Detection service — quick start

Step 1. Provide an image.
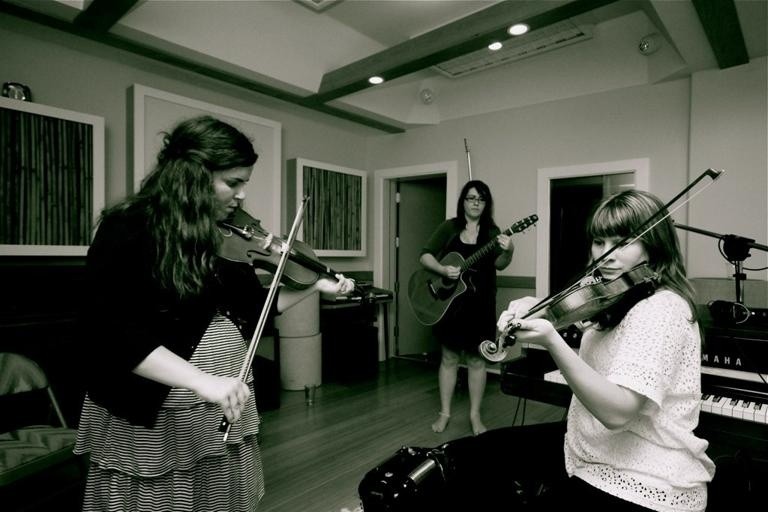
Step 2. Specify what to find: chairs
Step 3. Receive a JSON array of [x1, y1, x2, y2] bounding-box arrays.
[[1, 352, 76, 511]]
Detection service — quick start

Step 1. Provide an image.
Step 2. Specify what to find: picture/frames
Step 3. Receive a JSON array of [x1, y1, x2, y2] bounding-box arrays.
[[126, 85, 281, 238], [1, 97, 105, 255], [286, 157, 365, 255]]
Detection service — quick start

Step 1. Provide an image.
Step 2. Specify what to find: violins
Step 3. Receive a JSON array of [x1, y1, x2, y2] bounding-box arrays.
[[215, 205, 377, 313], [476, 260, 658, 365]]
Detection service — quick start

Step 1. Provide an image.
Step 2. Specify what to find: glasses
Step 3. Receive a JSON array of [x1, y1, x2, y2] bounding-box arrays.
[[465, 194, 487, 204]]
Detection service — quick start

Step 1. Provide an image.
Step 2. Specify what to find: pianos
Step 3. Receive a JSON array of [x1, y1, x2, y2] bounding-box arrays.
[[499, 301, 763, 496]]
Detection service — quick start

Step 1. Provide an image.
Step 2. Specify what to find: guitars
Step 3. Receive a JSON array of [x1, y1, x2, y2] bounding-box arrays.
[[406, 214, 538, 325]]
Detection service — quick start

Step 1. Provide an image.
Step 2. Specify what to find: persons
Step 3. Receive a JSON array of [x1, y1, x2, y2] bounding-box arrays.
[[446, 189, 719, 512], [418, 178, 514, 436], [70, 115, 355, 511]]
